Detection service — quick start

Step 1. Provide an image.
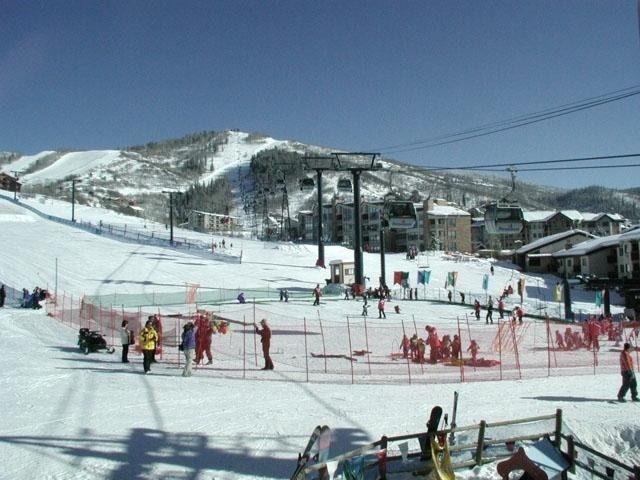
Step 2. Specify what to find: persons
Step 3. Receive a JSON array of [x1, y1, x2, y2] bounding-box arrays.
[[488, 295, 494, 308], [19, 286, 48, 310], [151, 317, 157, 331], [555, 312, 640, 352], [515, 306, 523, 323], [155, 314, 162, 354], [0, 284, 7, 307], [344, 283, 418, 319], [177, 309, 214, 377], [121, 320, 135, 363], [617, 343, 639, 402], [237, 292, 247, 303], [485, 303, 494, 325], [473, 301, 481, 321], [459, 291, 465, 304], [312, 283, 323, 306], [447, 290, 453, 304], [254, 318, 275, 370], [496, 296, 505, 319], [405, 245, 420, 260], [140, 320, 157, 374], [398, 325, 480, 364]]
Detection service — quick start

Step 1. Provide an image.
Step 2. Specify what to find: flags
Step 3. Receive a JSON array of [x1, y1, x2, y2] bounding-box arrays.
[[555, 284, 562, 303], [596, 290, 603, 307], [394, 270, 459, 287], [482, 274, 490, 289]]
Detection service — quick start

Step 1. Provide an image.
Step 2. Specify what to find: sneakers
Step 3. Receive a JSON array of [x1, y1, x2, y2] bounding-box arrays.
[[193, 358, 201, 363], [269, 365, 274, 369], [205, 360, 213, 365], [261, 366, 270, 370], [145, 369, 153, 375], [618, 397, 627, 403], [631, 397, 640, 402]]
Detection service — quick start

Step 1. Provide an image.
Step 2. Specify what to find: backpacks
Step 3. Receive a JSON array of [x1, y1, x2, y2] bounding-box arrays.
[[126, 328, 135, 345]]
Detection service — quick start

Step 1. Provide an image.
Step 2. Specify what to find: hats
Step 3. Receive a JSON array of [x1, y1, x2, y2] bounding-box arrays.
[[258, 319, 267, 323]]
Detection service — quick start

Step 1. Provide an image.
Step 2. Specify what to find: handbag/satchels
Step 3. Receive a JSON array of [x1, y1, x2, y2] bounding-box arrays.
[[179, 343, 183, 351]]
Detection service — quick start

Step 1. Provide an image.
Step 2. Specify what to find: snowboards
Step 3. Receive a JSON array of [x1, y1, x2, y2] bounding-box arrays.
[[291, 425, 331, 480], [413, 406, 442, 476]]
